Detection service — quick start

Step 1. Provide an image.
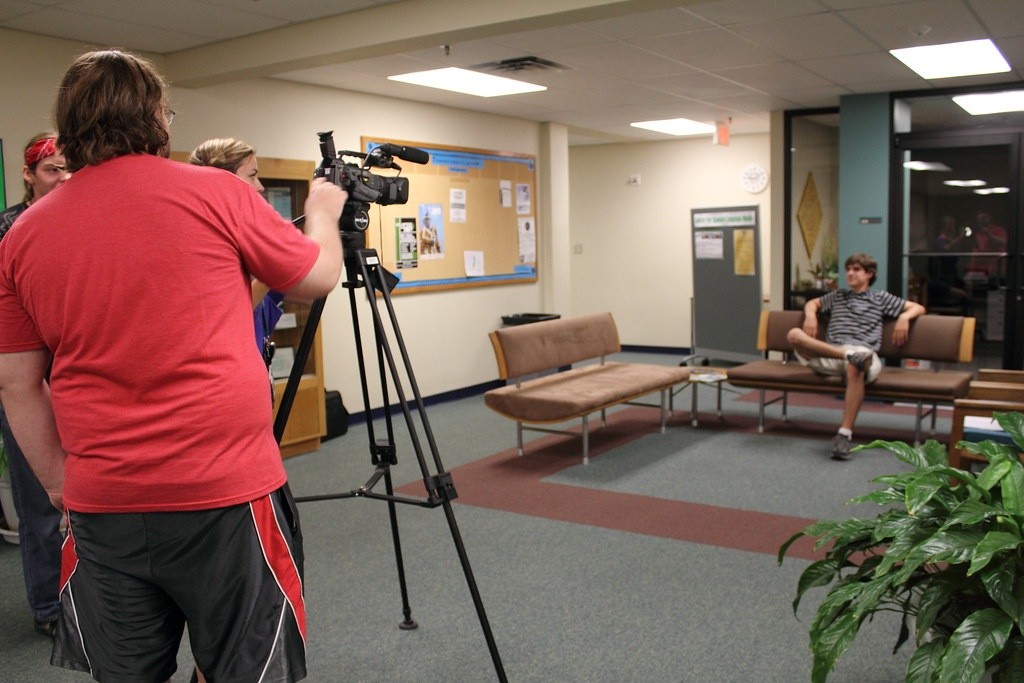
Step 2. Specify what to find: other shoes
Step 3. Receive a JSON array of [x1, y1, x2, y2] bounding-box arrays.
[[35, 615, 63, 637]]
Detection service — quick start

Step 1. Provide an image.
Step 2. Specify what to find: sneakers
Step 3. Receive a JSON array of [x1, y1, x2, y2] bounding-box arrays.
[[829, 431, 851, 460], [847, 349, 872, 373]]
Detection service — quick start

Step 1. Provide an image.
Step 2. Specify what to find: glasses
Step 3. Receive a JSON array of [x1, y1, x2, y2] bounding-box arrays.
[[159, 105, 176, 125]]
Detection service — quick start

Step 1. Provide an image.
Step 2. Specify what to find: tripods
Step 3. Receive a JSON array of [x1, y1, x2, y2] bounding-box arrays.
[[271, 200, 510, 683]]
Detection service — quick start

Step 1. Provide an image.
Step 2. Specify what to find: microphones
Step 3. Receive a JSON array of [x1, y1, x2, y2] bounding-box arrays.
[[380, 143, 429, 165]]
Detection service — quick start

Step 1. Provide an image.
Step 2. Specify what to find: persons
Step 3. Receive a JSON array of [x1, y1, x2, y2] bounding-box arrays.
[[915, 213, 1007, 307], [787, 254, 925, 459], [0, 51, 348, 682]]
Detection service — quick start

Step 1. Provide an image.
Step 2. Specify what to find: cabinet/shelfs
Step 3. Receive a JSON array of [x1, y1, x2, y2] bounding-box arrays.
[[170, 149, 328, 459]]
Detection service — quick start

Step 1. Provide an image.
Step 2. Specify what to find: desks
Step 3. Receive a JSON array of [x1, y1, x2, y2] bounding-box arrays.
[[949, 368, 1024, 485]]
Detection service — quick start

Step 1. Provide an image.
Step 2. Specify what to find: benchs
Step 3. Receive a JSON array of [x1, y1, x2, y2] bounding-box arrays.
[[727, 311, 975, 452], [486, 310, 691, 465]]
